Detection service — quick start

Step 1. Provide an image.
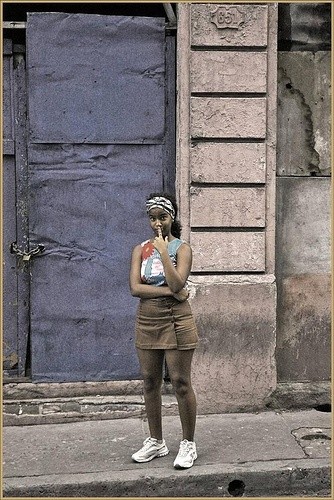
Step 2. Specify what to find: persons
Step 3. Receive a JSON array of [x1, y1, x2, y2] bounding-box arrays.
[[129, 191, 200, 470]]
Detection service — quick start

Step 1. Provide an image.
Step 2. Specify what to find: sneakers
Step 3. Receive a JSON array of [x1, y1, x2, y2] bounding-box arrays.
[[131, 437, 169, 463], [172, 439, 198, 469]]
